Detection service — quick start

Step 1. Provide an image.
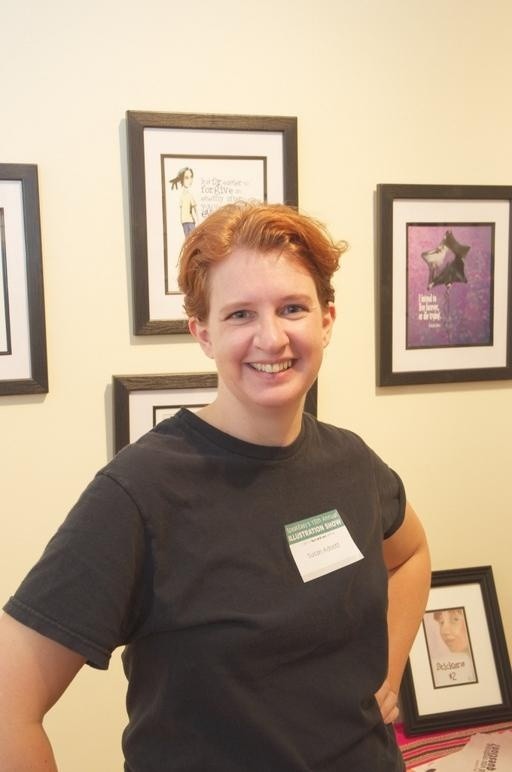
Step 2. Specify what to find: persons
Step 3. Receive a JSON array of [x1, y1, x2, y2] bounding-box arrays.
[[0, 196, 434, 772], [434, 608, 470, 653], [166, 163, 201, 237]]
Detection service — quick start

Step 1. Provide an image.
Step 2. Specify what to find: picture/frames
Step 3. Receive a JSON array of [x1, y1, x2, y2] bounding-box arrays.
[[374, 184, 510, 392], [1, 161, 49, 398], [125, 110, 301, 339], [113, 370, 319, 461], [396, 563, 512, 735]]
